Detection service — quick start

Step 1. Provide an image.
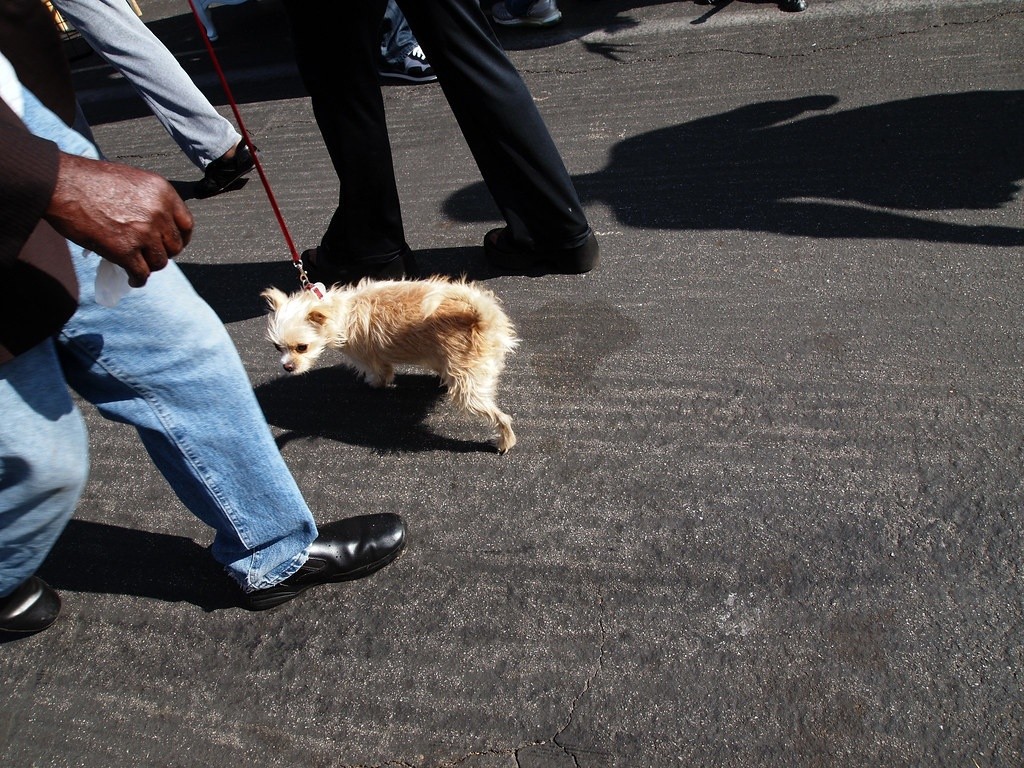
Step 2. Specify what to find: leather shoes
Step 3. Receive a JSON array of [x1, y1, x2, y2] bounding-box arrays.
[[192, 139, 265, 196], [230, 513, 406, 609], [0, 576, 63, 634]]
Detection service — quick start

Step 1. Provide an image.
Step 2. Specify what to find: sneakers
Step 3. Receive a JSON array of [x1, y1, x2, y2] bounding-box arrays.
[[378, 44, 438, 82], [491, 0, 561, 26]]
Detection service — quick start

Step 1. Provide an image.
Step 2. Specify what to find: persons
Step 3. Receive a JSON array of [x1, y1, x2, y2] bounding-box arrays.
[[0, 0, 408, 639], [284, 0, 599, 282], [490, 0, 562, 26], [377, 0, 437, 83], [50, 0, 259, 199]]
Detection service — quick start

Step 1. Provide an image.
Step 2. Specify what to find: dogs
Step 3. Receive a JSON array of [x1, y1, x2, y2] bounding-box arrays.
[[260, 271, 518, 454]]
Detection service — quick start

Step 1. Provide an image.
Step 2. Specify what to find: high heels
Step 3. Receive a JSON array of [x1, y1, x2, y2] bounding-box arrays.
[[484, 226, 601, 275], [302, 243, 416, 281]]
